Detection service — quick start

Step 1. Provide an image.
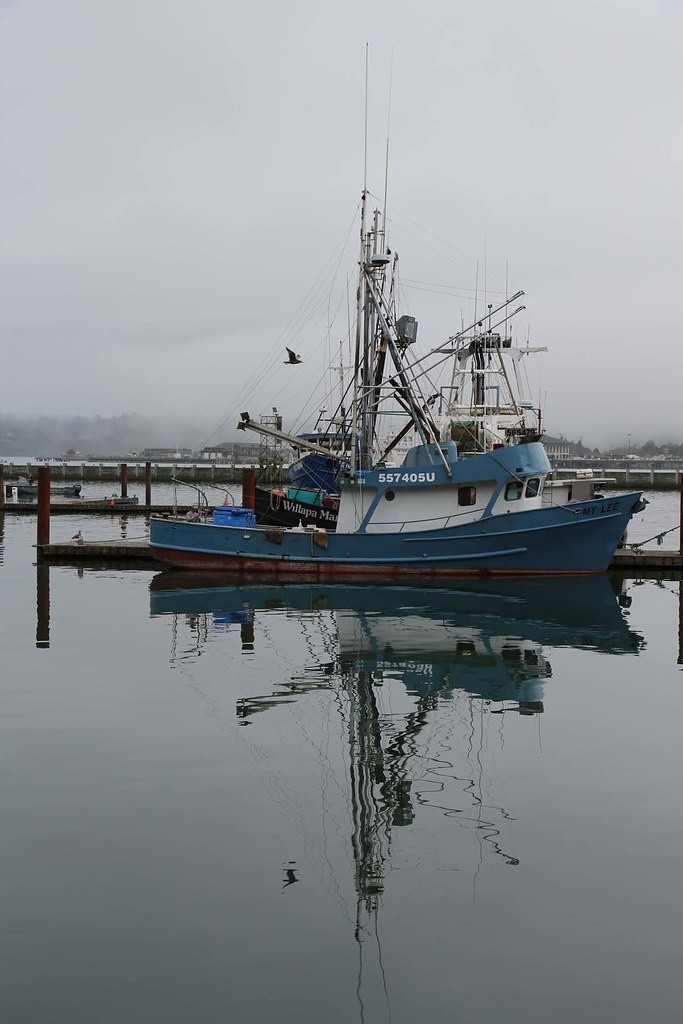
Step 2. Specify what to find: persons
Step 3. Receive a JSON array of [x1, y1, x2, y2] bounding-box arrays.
[[186, 504, 201, 523]]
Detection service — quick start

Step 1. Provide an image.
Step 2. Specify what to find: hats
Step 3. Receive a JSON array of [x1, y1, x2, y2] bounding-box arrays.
[[193, 504, 198, 509]]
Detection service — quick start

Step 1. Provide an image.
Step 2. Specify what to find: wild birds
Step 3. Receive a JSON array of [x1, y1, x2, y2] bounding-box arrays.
[[284, 346, 303, 364], [72, 529, 83, 540]]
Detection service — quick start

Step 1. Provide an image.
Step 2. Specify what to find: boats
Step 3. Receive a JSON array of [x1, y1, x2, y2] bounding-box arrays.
[[5, 481, 82, 496]]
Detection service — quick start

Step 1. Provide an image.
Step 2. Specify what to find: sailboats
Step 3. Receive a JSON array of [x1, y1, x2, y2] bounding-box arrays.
[[150, 150, 648, 586], [145, 568, 645, 954]]
